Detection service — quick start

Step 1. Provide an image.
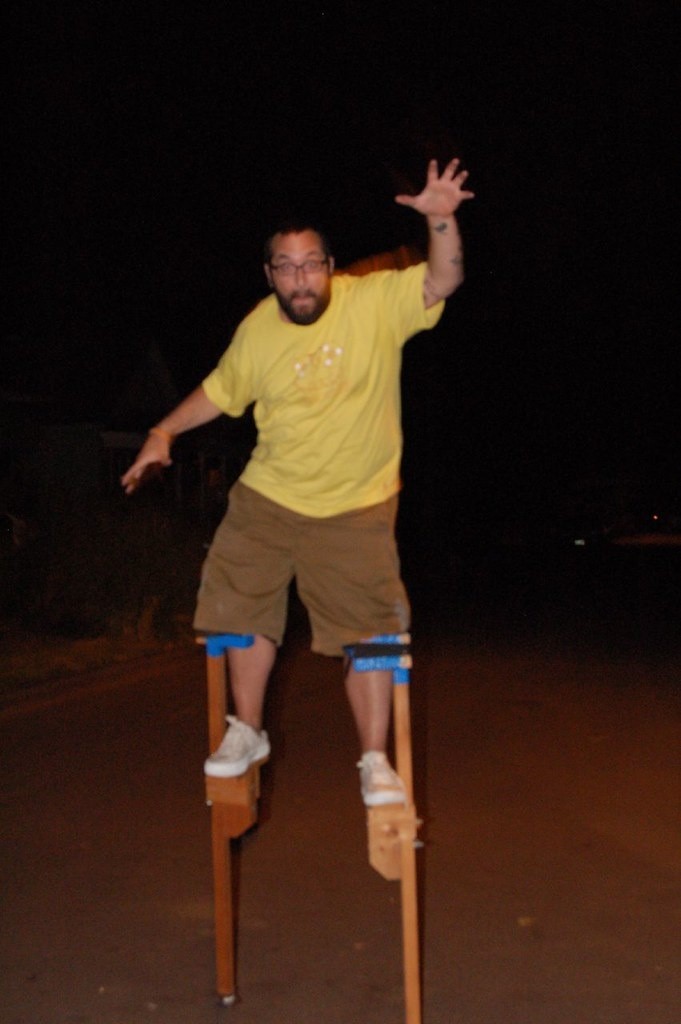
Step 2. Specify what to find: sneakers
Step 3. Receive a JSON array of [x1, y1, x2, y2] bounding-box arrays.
[[356, 751, 406, 807], [204, 715, 271, 778]]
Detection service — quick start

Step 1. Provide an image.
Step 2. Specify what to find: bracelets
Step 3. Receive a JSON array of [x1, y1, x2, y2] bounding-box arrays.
[[149, 427, 174, 445]]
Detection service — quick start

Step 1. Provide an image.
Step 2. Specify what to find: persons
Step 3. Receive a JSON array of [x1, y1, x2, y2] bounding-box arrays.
[[120, 157, 476, 806]]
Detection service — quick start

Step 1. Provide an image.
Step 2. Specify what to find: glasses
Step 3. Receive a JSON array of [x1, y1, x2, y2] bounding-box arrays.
[[269, 258, 327, 275]]
